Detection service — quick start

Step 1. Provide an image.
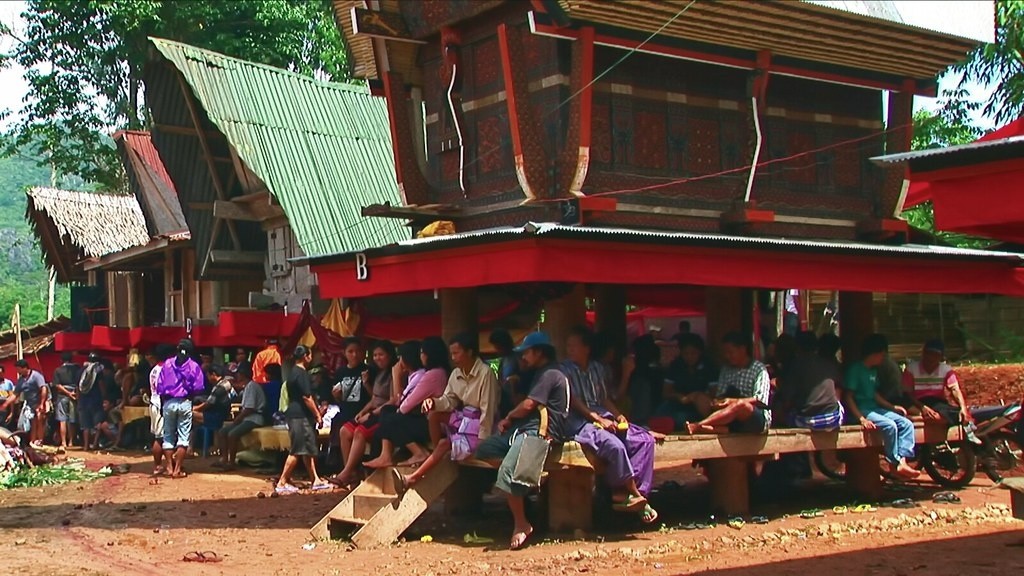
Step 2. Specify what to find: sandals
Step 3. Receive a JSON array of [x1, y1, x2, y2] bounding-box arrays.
[[510, 524, 534, 549]]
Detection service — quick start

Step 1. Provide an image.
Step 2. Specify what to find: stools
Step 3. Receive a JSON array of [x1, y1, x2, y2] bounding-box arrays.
[[193, 424, 222, 459]]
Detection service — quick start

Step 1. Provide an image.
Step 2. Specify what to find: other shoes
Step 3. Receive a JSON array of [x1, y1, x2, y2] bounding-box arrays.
[[154, 465, 164, 475], [211, 459, 239, 471]]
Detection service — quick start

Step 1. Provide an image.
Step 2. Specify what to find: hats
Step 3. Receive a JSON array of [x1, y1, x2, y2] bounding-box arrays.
[[924, 339, 945, 355], [180, 339, 191, 347], [89, 352, 98, 357], [513, 332, 551, 352]]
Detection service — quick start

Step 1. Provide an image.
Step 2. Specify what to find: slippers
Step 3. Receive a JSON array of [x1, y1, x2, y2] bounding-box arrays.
[[800, 508, 824, 519], [392, 468, 406, 500], [163, 471, 172, 477], [172, 472, 187, 478], [933, 492, 962, 505], [727, 515, 768, 526], [833, 506, 847, 514], [851, 504, 877, 513], [673, 521, 697, 529], [698, 523, 716, 528], [612, 494, 647, 511], [311, 481, 331, 490], [636, 503, 658, 522], [327, 477, 350, 491], [276, 483, 298, 493], [892, 498, 914, 508]]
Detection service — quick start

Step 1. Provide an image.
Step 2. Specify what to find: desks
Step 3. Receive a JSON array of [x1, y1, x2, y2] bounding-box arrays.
[[223, 421, 331, 453], [122, 406, 149, 425]]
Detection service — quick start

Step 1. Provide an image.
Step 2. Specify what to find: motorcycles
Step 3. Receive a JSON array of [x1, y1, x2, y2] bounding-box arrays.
[[814, 398, 1024, 489]]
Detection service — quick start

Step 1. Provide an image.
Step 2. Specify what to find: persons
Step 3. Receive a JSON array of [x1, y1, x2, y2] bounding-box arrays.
[[156, 339, 206, 478], [275, 344, 330, 492], [476, 331, 571, 551], [0, 321, 984, 493], [555, 325, 658, 524]]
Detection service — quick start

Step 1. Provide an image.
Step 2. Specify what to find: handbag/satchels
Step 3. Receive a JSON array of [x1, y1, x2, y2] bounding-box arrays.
[[511, 436, 551, 487]]
